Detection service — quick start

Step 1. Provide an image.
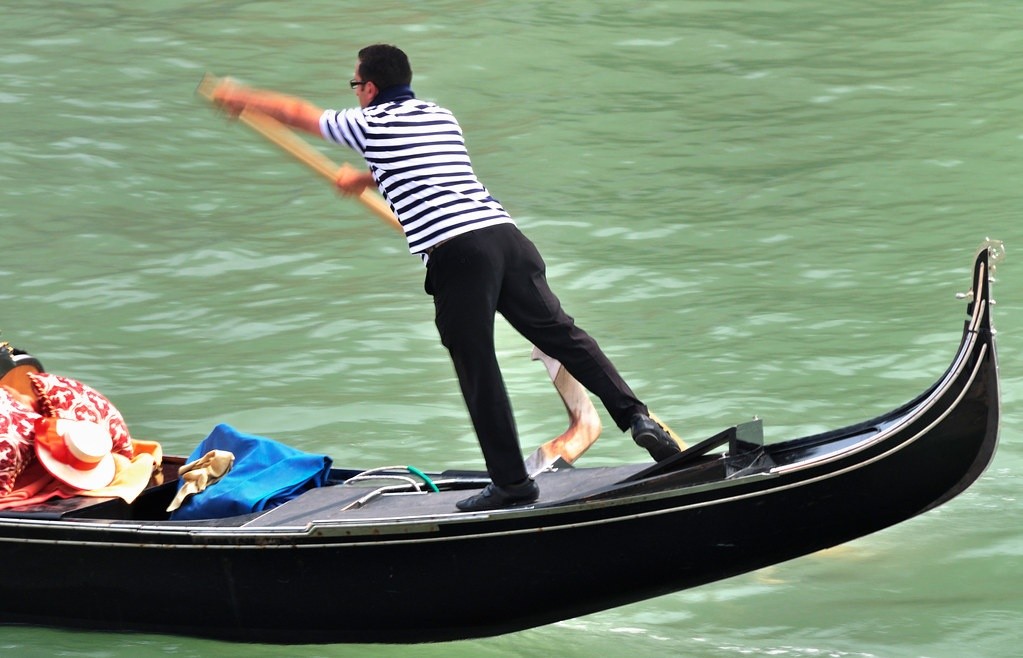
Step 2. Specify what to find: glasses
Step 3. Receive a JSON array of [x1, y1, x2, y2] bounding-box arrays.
[[349, 78, 367, 90]]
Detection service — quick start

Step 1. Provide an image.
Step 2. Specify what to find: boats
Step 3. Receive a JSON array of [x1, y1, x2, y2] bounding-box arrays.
[[0, 234, 1005, 646]]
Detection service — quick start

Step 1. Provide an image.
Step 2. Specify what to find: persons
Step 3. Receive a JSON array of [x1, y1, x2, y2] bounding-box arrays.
[[215, 44, 687, 511]]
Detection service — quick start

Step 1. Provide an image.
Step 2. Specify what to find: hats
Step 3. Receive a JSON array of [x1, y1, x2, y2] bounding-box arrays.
[[35, 418, 115, 490]]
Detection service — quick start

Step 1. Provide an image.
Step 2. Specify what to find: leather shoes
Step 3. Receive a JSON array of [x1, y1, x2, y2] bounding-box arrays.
[[630, 414, 681, 463], [456, 479, 539, 511]]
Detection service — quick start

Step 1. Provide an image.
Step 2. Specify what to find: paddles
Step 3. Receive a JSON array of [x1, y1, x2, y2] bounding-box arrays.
[[191, 67, 689, 453]]
